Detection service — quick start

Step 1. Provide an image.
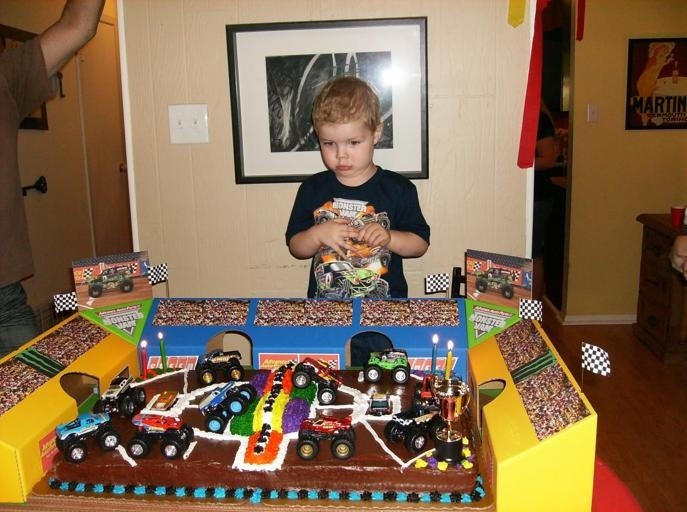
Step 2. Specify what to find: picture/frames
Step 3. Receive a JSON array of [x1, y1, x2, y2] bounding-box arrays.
[[1, 24, 50, 131], [621, 35, 687, 131], [222, 13, 431, 187]]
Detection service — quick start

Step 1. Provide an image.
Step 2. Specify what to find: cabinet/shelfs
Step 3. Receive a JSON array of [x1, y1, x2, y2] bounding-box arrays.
[[630, 213, 687, 367]]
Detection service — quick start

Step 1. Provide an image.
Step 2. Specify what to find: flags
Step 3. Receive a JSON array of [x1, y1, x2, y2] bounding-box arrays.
[[147, 263, 168, 285], [426, 273, 449, 292], [582, 342, 611, 378], [519, 298, 543, 322], [54, 292, 78, 313]]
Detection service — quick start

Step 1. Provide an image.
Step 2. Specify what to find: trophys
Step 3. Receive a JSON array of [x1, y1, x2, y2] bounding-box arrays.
[[431, 375, 471, 462]]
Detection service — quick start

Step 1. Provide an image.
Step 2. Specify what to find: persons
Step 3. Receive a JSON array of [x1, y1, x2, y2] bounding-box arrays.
[[533, 109, 556, 300], [286, 76, 430, 298]]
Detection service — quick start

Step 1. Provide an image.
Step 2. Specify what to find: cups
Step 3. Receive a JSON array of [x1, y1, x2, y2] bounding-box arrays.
[[670, 205, 685, 229]]
